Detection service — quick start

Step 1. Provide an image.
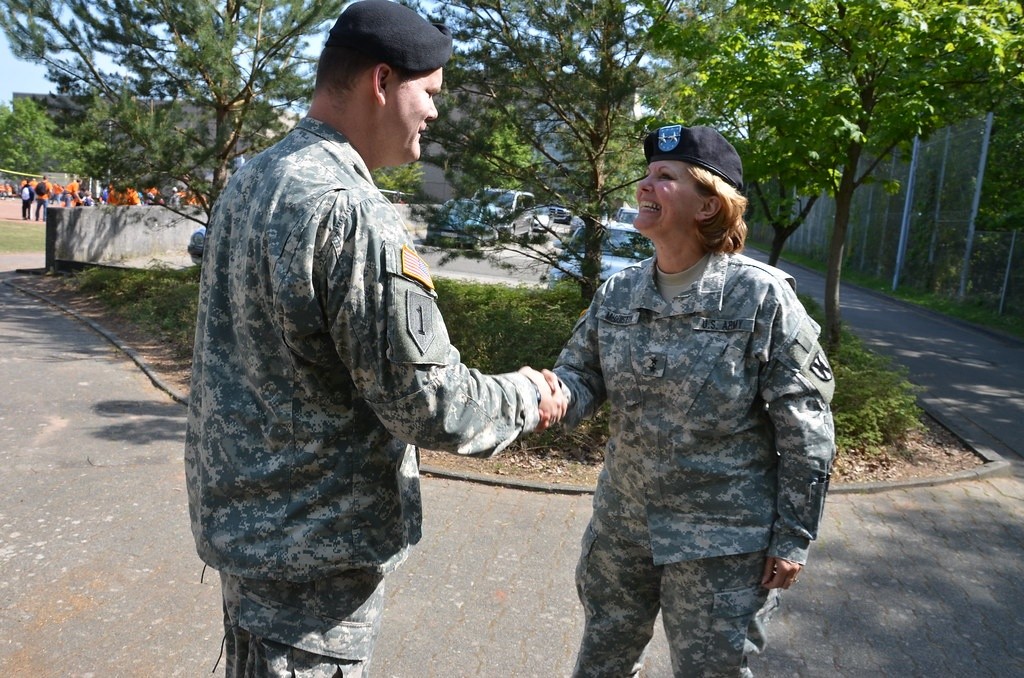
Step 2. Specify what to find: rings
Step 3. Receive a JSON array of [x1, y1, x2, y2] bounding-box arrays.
[[793, 577, 799, 583]]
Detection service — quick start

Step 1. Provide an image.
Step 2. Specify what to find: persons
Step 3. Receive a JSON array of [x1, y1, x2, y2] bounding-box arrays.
[[0, 172, 210, 223], [183, 0, 561, 678], [533, 124, 837, 678]]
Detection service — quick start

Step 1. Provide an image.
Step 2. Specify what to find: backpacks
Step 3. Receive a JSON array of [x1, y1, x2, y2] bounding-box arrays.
[[21, 187, 29, 200], [35, 182, 47, 195]]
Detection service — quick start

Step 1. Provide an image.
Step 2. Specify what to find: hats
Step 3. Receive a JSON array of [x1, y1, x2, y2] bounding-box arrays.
[[643, 125, 743, 190], [325, 0, 453, 72]]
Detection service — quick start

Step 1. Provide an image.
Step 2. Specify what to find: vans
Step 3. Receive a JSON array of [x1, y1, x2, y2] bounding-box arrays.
[[471, 187, 536, 242]]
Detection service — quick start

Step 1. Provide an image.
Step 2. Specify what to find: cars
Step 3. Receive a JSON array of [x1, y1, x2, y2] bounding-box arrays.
[[569, 212, 611, 236], [188, 228, 207, 265], [616, 207, 639, 225], [553, 212, 572, 225], [426, 199, 498, 250], [547, 222, 655, 291], [533, 205, 554, 233]]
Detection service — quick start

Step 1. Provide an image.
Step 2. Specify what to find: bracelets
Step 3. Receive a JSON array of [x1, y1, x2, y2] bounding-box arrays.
[[558, 378, 563, 391], [523, 374, 543, 408]]
